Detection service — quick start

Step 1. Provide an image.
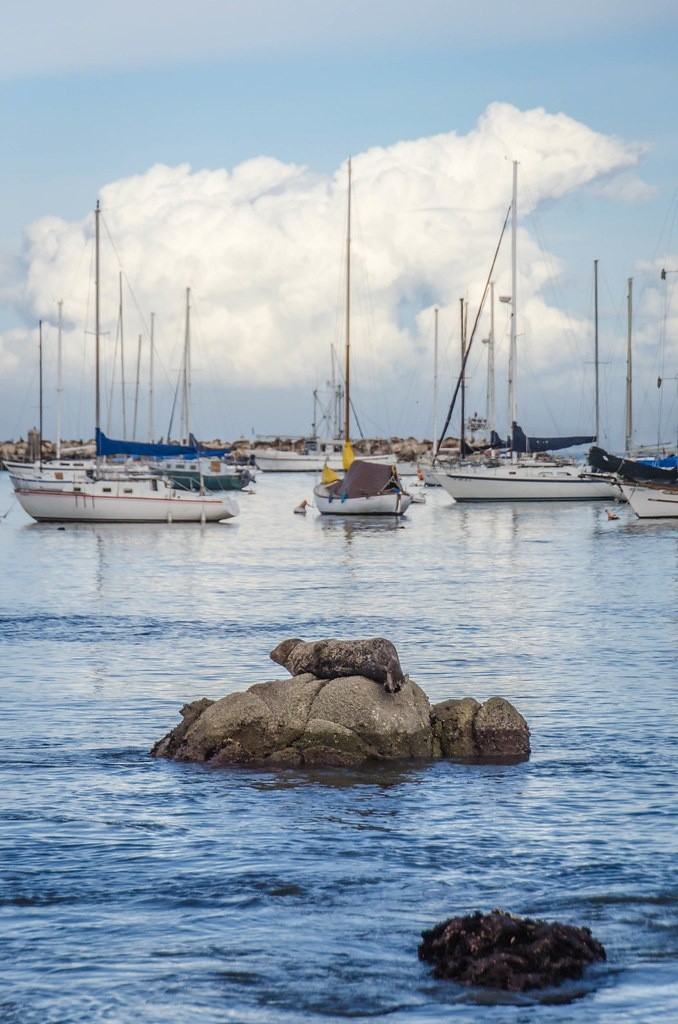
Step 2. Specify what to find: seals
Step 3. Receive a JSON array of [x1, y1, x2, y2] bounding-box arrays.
[[269, 638, 405, 693]]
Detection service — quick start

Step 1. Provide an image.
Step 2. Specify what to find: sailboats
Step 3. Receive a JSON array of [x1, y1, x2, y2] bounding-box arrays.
[[1, 152, 678, 519]]
